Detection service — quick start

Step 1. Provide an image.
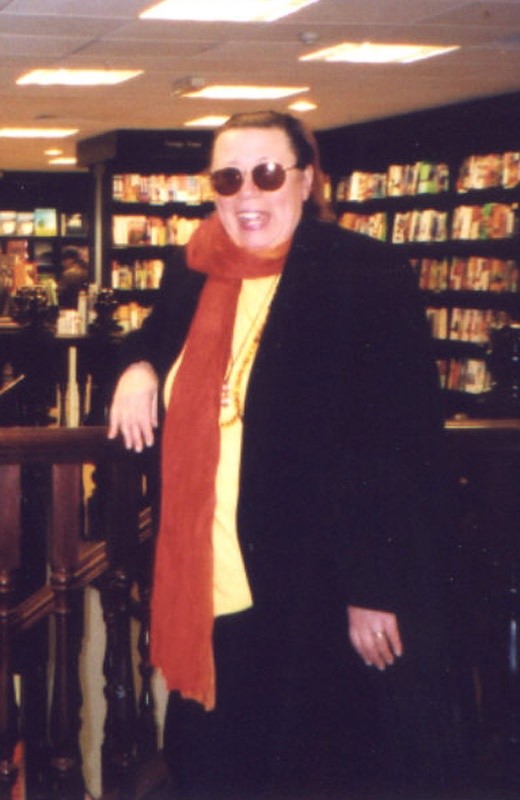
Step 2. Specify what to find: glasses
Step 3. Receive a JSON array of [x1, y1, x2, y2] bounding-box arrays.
[[206, 161, 299, 197]]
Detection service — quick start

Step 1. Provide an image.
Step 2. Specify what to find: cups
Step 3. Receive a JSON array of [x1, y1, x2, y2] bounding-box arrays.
[[0, 253, 19, 323]]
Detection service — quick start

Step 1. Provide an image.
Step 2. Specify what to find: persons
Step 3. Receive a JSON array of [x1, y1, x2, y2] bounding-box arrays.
[[58, 108, 456, 799]]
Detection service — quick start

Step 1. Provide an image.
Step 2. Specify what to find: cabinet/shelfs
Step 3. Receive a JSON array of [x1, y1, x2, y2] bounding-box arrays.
[[0, 97, 520, 418]]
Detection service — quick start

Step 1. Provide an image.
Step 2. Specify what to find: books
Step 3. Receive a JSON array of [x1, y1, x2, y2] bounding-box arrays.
[[0, 149, 520, 395]]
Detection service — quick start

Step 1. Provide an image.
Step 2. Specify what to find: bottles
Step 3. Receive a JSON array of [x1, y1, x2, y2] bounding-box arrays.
[[36, 263, 58, 305], [7, 240, 33, 289]]
